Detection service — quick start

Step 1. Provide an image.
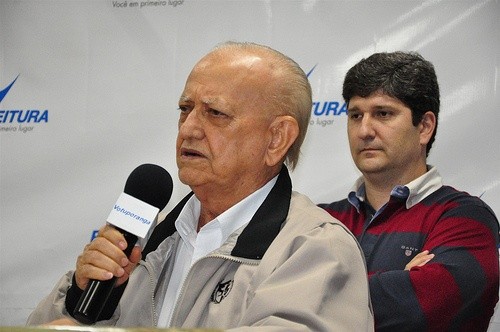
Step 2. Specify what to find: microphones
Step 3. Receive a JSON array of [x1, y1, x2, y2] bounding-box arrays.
[[72, 164, 175, 326]]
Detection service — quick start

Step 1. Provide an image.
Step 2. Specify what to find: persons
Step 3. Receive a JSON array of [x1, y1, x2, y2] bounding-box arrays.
[[27, 39, 376, 332], [316, 49, 500, 331]]
